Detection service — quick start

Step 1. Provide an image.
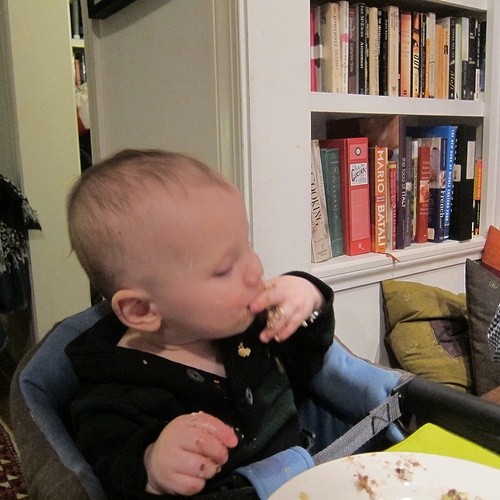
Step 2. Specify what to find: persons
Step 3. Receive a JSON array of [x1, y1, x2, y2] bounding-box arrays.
[[0, 173, 43, 387], [65, 146, 336, 499]]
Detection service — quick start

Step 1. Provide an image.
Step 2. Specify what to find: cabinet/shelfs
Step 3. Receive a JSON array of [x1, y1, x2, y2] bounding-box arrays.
[[0, 0, 93, 342], [81, 0, 500, 369]]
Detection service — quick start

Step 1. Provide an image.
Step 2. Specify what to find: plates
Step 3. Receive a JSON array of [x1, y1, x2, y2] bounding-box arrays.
[[267, 450, 500, 500]]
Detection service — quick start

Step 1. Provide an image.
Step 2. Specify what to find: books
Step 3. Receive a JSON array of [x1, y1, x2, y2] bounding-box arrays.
[[310, 1, 488, 102], [311, 114, 481, 264]]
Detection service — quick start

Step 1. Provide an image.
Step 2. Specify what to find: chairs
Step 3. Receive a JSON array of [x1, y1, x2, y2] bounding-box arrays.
[[8, 298, 500, 500]]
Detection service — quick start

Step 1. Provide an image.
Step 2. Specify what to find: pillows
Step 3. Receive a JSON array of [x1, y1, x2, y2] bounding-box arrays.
[[378, 226, 500, 398]]
